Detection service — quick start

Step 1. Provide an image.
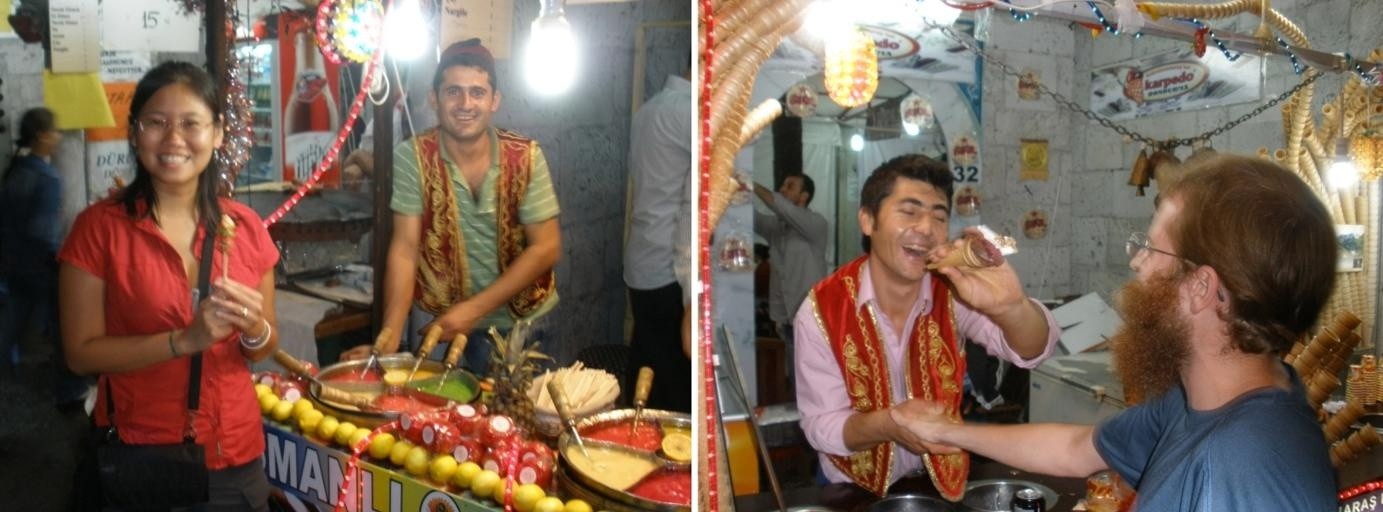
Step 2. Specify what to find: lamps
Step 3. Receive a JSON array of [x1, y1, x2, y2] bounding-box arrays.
[[1323, 72, 1360, 192]]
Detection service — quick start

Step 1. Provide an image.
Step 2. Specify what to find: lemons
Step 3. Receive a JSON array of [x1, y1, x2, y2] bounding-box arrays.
[[254, 384, 592, 512]]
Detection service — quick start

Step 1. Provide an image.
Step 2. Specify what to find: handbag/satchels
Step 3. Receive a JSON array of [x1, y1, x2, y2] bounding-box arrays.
[[82, 440, 208, 512]]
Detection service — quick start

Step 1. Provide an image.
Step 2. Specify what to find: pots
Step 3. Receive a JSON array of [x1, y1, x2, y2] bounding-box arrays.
[[308, 355, 481, 417], [558, 407, 690, 510]]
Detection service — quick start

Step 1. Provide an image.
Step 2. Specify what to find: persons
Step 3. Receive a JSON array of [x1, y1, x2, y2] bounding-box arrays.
[[619, 41, 692, 422], [883, 151, 1351, 511], [788, 150, 1067, 512], [732, 164, 830, 391], [753, 238, 782, 342], [56, 57, 287, 511], [669, 161, 692, 365], [334, 35, 565, 395], [5, 103, 90, 424]]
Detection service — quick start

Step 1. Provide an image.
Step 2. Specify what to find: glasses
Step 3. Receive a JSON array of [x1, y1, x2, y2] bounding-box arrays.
[[1126, 231, 1226, 304], [131, 114, 216, 135]]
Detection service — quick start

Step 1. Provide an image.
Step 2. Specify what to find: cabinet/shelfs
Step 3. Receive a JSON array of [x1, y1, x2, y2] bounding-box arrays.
[[235, 36, 282, 184]]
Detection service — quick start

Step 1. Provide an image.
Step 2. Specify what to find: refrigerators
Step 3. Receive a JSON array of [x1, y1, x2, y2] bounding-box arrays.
[[1028, 347, 1137, 425], [223, 12, 340, 188]]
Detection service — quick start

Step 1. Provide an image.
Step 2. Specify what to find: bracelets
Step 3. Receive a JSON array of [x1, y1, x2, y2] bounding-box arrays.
[[240, 318, 268, 344], [238, 318, 273, 351], [163, 329, 184, 363]]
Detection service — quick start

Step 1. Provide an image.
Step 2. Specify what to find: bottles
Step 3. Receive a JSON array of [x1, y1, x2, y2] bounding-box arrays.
[[232, 50, 277, 184], [282, 28, 342, 180]]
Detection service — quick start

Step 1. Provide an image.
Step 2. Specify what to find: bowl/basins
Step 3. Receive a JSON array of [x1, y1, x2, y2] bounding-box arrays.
[[527, 369, 621, 449], [868, 478, 1059, 512]]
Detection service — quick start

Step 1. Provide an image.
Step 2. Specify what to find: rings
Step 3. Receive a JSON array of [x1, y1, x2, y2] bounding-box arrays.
[[239, 306, 250, 319]]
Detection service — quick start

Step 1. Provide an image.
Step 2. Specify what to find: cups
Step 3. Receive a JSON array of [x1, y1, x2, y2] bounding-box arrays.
[[1282, 307, 1382, 467], [1344, 353, 1381, 408]]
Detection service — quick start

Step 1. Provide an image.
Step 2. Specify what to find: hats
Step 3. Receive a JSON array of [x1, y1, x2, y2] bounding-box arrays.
[[441, 39, 494, 64]]
